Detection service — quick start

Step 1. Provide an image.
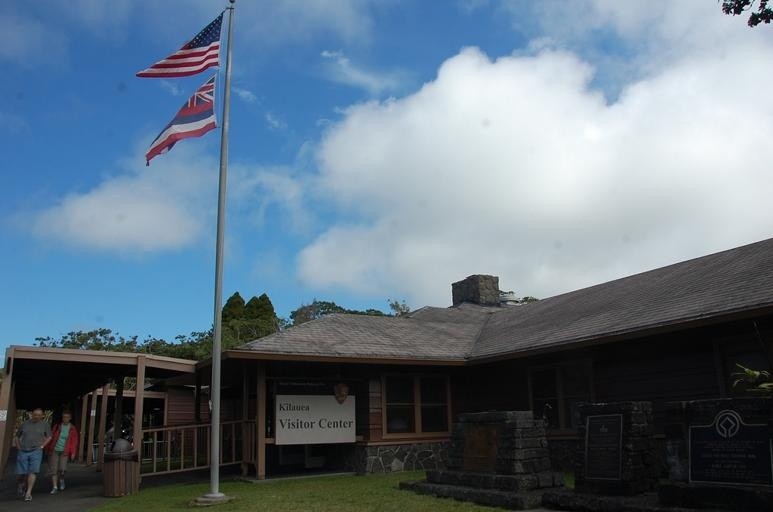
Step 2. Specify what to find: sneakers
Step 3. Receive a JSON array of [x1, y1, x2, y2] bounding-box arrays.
[[50, 485, 65, 494], [17, 481, 32, 501]]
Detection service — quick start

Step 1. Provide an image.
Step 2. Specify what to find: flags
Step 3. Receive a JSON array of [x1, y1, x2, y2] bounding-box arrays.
[[144, 74, 217, 166], [136, 10, 224, 77]]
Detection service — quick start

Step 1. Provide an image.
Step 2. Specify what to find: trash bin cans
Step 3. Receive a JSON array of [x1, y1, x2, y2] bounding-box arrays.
[[105, 438, 138, 497]]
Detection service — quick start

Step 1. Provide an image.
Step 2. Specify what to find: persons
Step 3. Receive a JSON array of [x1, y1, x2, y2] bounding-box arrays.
[[50, 410, 78, 495], [14, 408, 52, 501]]
